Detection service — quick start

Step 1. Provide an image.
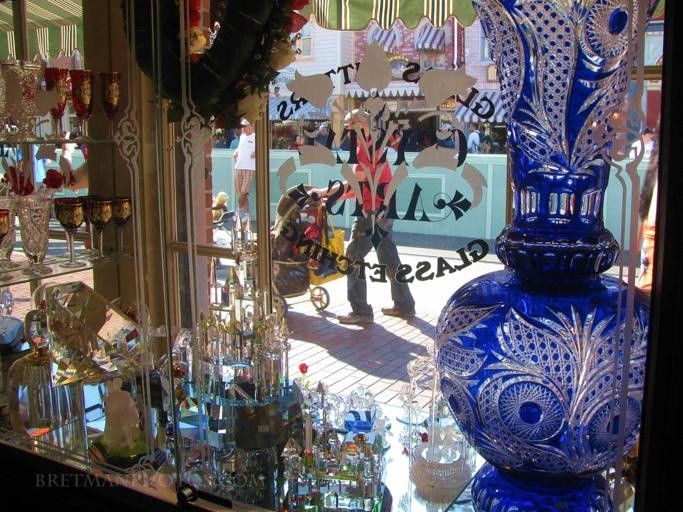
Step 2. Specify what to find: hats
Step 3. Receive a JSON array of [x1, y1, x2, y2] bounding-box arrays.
[[240, 119, 249, 126]]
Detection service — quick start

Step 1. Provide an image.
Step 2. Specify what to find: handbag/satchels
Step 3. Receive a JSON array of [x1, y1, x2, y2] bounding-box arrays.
[[313, 256, 336, 277], [310, 206, 346, 286]]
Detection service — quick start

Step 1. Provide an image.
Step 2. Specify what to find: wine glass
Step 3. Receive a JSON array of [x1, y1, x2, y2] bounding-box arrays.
[[0, 68, 12, 141], [16, 196, 51, 273], [101, 72, 120, 139], [70, 69, 94, 140], [46, 67, 68, 140], [12, 68, 42, 141], [113, 199, 131, 260], [87, 199, 110, 262], [54, 197, 85, 268], [0, 196, 17, 280]]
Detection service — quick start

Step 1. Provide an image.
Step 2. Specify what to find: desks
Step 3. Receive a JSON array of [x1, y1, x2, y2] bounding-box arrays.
[[0, 404, 483, 512]]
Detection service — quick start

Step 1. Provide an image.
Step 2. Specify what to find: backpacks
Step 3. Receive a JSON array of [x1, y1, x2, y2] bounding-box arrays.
[[473, 131, 491, 152]]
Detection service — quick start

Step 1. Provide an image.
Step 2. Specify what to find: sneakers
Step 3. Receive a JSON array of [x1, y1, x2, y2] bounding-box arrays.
[[337, 313, 375, 324], [241, 216, 249, 223], [381, 307, 414, 318]]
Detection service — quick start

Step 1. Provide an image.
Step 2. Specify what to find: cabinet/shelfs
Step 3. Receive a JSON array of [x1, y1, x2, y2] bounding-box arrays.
[[0, 1, 134, 438]]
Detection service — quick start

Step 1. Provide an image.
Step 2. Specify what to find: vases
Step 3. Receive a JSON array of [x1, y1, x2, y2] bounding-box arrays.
[[434, 0, 650, 512]]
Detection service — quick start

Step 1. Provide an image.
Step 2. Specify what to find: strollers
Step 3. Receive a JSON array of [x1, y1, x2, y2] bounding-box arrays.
[[230, 186, 346, 312]]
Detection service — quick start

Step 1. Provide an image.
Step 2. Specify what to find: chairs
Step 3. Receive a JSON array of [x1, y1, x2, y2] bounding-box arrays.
[[213, 210, 235, 238]]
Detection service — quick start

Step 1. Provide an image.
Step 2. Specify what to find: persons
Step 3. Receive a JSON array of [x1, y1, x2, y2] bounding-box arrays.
[[59, 132, 87, 189], [629, 127, 654, 162], [213, 133, 226, 148], [232, 119, 255, 224], [287, 122, 329, 150], [69, 126, 81, 139], [466, 122, 483, 153], [230, 129, 242, 149], [307, 108, 415, 324]]
